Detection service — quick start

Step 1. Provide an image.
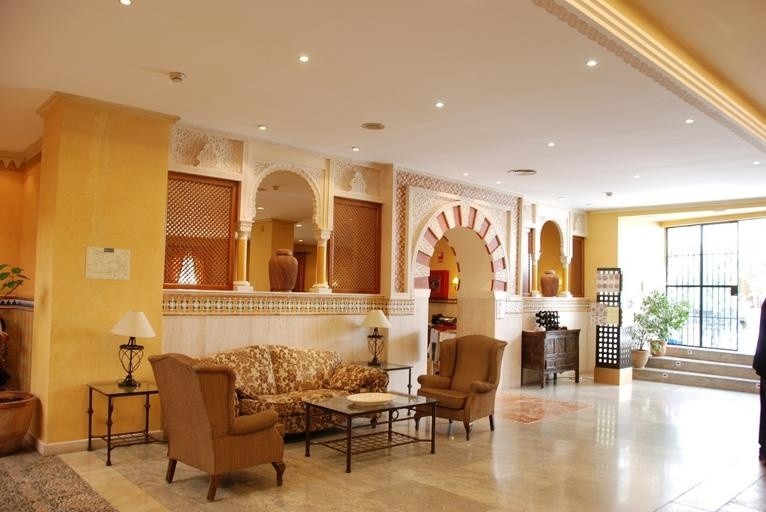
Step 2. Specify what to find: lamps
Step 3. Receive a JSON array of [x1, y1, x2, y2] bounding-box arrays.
[[361, 308, 392, 367], [110, 309, 157, 387], [452, 277, 459, 290]]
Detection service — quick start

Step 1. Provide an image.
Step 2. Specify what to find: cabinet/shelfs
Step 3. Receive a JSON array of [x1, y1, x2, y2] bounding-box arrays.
[[521, 328, 582, 387]]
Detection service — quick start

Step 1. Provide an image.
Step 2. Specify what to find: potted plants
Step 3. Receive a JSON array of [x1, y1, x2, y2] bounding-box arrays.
[[626, 327, 655, 368], [636, 292, 691, 357], [0, 264, 38, 457]]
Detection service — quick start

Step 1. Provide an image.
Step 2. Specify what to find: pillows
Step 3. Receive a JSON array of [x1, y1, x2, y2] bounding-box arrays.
[[323, 367, 370, 391], [236, 381, 257, 400]]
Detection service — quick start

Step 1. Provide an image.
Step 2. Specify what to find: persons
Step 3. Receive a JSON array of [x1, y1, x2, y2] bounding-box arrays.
[[753, 298, 766, 458]]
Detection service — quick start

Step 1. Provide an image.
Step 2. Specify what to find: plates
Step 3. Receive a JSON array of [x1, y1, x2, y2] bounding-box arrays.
[[347, 392, 397, 407]]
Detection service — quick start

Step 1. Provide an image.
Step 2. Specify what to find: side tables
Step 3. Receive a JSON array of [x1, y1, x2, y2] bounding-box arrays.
[[350, 362, 415, 416], [86, 379, 159, 465]]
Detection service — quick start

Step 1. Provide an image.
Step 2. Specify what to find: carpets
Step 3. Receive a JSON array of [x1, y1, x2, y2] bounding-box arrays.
[[496, 389, 593, 425]]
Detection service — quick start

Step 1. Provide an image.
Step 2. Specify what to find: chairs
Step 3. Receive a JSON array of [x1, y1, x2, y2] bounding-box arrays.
[[147, 353, 286, 500], [415, 334, 508, 440]]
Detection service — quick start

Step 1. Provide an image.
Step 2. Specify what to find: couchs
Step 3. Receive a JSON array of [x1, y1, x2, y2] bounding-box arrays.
[[199, 345, 389, 435]]
